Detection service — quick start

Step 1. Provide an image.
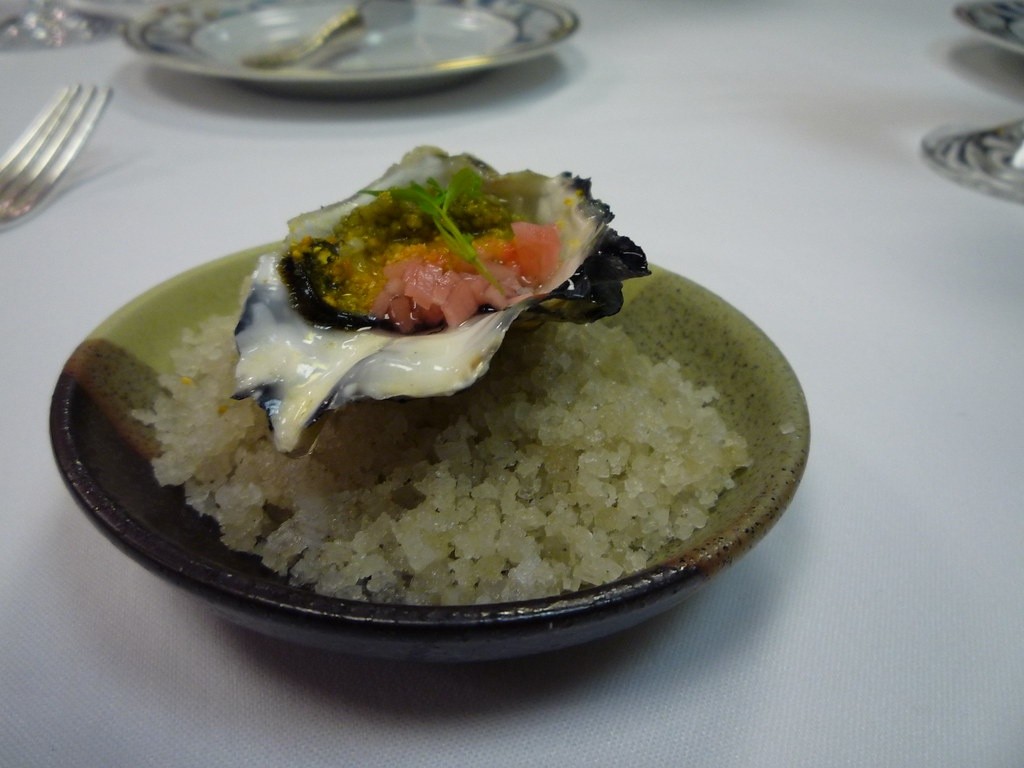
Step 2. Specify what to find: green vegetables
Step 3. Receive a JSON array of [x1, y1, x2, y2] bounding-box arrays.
[[357, 169, 508, 298]]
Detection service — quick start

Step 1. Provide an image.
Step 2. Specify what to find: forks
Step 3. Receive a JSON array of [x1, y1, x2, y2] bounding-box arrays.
[[1, 84, 111, 224]]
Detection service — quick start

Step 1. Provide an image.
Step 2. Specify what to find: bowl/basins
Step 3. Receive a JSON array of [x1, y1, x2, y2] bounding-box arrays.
[[49, 237, 809, 666]]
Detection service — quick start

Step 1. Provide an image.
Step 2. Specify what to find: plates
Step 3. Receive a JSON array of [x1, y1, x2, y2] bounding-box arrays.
[[952, 0, 1024, 53], [123, 1, 581, 99]]
